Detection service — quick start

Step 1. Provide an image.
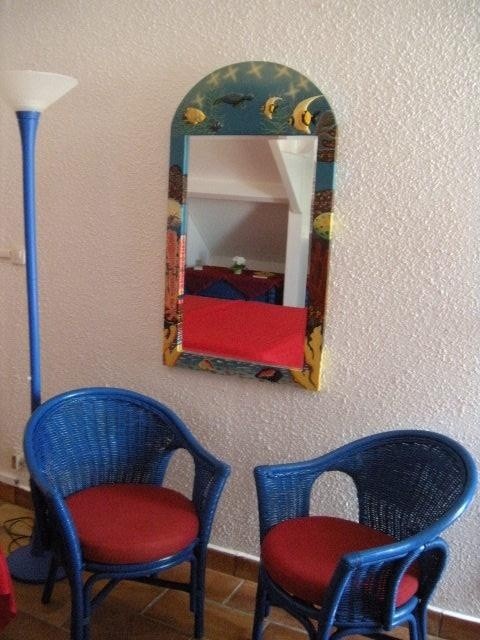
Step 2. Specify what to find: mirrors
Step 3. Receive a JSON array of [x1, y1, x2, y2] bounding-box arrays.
[[160, 59, 340, 393]]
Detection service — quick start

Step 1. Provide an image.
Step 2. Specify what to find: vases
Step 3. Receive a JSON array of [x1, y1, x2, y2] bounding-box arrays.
[[231, 269, 242, 275]]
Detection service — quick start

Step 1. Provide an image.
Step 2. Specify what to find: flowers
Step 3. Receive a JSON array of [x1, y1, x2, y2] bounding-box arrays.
[[230, 255, 248, 269]]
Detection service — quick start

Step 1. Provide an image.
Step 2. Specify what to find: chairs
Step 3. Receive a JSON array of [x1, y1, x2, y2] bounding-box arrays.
[[21, 384, 230, 638], [251, 428, 478, 638]]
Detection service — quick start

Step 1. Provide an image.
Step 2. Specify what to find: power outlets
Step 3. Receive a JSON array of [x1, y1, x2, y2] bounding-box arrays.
[[14, 445, 24, 472]]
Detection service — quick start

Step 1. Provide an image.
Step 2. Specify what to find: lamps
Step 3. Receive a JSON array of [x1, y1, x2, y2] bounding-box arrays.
[[1, 68, 81, 583]]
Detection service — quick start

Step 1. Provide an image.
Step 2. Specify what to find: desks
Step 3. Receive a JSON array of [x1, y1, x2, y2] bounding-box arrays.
[[186, 264, 284, 304]]
